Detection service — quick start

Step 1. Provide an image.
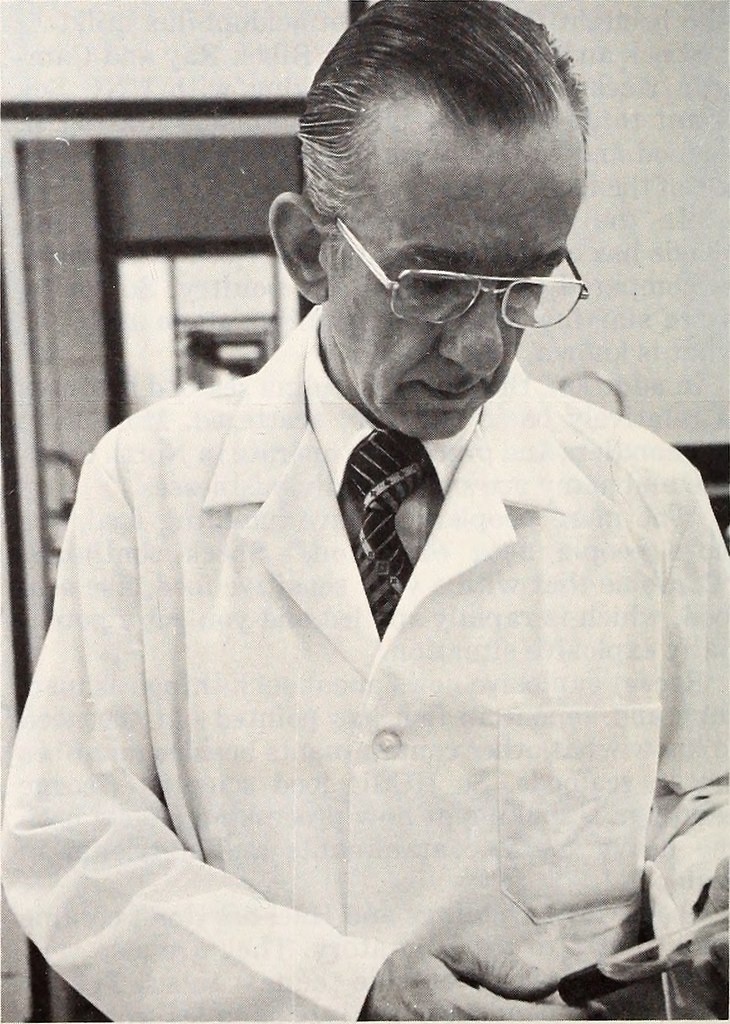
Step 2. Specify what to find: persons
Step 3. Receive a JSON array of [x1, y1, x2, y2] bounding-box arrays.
[[1, 0, 730, 1022], [181, 328, 238, 390]]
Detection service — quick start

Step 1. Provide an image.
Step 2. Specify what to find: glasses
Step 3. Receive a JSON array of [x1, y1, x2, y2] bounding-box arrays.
[[333, 216, 589, 330]]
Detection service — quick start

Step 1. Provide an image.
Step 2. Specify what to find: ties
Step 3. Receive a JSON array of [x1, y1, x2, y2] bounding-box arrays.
[[344, 428, 434, 641]]
